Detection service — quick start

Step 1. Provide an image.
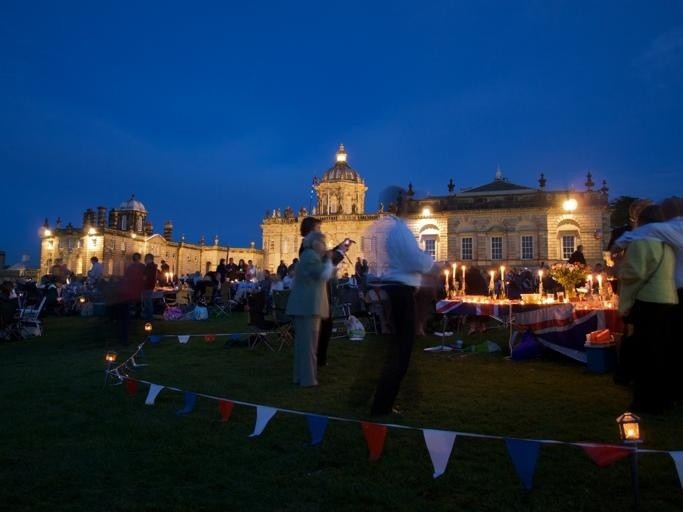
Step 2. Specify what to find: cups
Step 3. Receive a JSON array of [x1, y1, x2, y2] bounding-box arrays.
[[557, 291, 564, 302], [546, 294, 554, 304]]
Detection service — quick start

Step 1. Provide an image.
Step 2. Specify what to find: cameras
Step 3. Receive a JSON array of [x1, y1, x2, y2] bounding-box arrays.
[[344, 240, 351, 246]]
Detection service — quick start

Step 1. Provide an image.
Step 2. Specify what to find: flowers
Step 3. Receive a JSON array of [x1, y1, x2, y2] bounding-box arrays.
[[546, 255, 592, 289]]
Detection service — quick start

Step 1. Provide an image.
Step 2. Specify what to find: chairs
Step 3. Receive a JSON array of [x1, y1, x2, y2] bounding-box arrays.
[[16, 295, 49, 338], [247, 287, 295, 354], [214, 287, 232, 318]]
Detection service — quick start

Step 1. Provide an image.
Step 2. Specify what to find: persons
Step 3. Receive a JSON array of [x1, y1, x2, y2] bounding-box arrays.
[[155, 258, 299, 323], [348, 189, 434, 416], [331, 257, 373, 318], [123, 253, 145, 319], [104, 250, 139, 345], [41, 257, 104, 316], [415, 245, 620, 338], [285, 231, 334, 387], [142, 254, 158, 323], [299, 217, 355, 367], [609, 195, 681, 417]]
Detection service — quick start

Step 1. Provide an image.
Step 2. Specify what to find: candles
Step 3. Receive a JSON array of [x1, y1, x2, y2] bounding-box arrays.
[[598, 275, 603, 289], [539, 271, 543, 282], [443, 261, 466, 287], [166, 274, 173, 283], [487, 265, 504, 287]]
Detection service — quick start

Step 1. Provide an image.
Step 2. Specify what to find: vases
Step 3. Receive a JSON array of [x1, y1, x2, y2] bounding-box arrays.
[[564, 283, 577, 300]]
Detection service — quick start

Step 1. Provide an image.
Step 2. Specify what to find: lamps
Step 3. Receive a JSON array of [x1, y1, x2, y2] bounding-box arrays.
[[616, 409, 644, 511]]
